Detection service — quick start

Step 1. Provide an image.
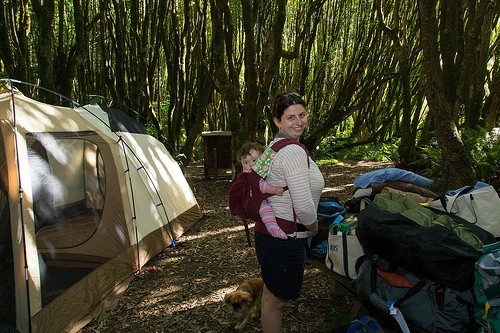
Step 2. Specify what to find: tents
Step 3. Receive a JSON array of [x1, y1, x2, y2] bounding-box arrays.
[[0, 79, 204, 333]]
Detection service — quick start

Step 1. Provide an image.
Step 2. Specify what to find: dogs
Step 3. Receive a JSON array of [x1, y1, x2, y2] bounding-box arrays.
[[224, 278, 265, 330]]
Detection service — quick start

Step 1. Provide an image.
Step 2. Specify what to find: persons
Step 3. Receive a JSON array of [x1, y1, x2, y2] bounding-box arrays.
[[254, 93, 325, 333], [239, 142, 288, 239]]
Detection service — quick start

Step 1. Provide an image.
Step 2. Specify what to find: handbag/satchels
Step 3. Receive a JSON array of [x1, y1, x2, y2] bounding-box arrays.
[[306, 168, 500, 333]]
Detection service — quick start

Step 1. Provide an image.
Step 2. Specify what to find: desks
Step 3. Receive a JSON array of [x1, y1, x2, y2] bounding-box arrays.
[[307, 260, 430, 333]]
